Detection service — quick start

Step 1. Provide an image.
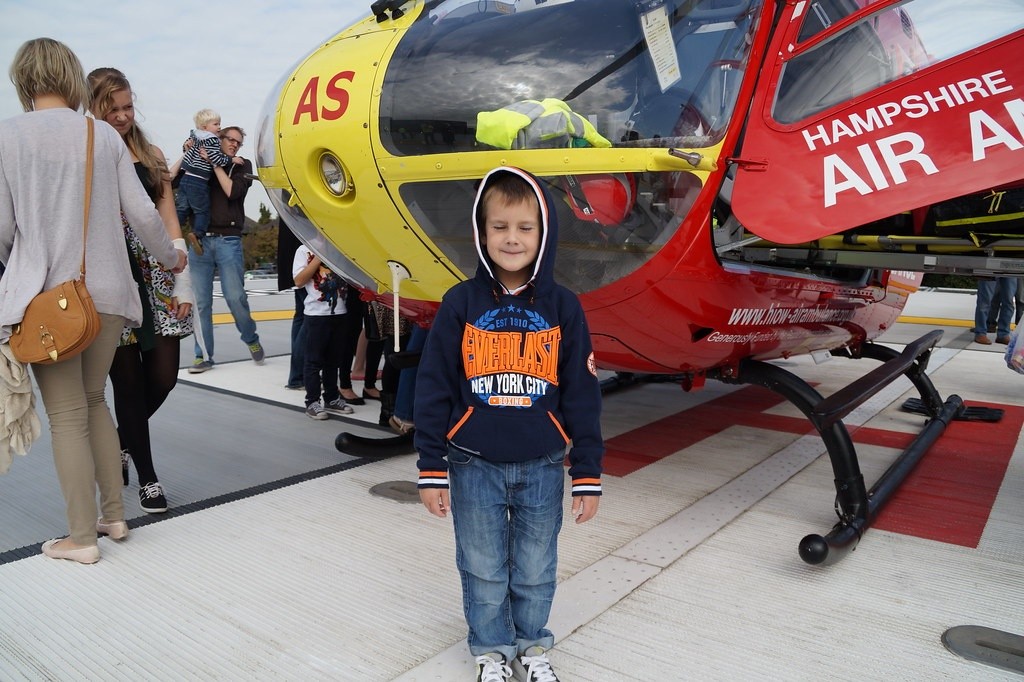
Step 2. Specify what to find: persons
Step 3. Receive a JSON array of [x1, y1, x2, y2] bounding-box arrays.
[[968, 277, 1024, 344], [414, 166, 602, 682], [0, 38, 186, 562], [84, 66, 194, 512], [170, 109, 265, 374], [278, 213, 429, 432]]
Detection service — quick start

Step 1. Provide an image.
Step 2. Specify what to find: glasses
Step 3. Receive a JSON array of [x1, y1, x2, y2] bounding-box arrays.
[[223, 135, 244, 148]]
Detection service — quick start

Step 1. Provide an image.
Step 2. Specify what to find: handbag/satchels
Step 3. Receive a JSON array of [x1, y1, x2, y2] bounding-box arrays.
[[9, 282, 99, 366], [1004, 312, 1024, 375]]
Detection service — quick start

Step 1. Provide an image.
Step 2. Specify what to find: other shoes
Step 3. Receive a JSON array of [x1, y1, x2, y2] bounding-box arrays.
[[994, 336, 1012, 344], [41, 537, 100, 564], [376, 369, 383, 380], [976, 334, 993, 345], [362, 388, 381, 400], [340, 391, 365, 405], [351, 372, 366, 380], [970, 325, 996, 333], [188, 232, 204, 256], [96, 517, 129, 538]]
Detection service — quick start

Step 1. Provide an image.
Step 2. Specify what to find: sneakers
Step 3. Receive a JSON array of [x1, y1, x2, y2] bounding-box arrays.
[[248, 342, 264, 364], [120, 449, 133, 486], [188, 357, 212, 373], [475, 648, 513, 682], [137, 483, 168, 513], [324, 395, 354, 414], [305, 401, 329, 420], [509, 644, 559, 682]]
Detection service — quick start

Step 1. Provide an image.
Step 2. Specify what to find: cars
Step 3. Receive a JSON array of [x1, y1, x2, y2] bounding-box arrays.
[[244, 262, 278, 280]]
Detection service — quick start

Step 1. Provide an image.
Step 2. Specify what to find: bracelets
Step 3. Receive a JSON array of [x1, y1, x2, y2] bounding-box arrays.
[[171, 238, 187, 254]]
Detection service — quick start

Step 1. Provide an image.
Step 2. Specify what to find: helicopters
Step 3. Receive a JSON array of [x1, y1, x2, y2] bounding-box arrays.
[[242, 0, 1024, 565]]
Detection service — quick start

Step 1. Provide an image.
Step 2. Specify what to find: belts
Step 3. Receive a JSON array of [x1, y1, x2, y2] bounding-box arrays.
[[205, 232, 220, 237]]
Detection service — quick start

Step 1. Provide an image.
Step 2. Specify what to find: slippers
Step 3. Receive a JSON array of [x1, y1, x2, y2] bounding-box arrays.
[[390, 415, 413, 435]]
